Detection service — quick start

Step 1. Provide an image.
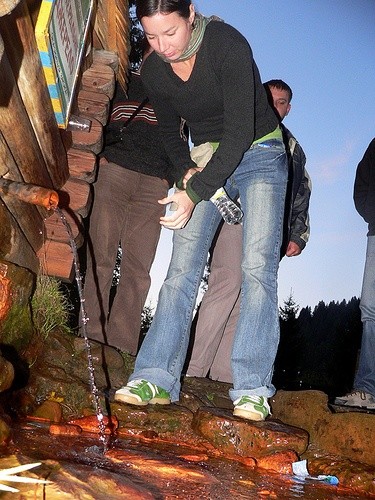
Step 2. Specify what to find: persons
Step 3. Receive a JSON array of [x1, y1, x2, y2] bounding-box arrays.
[[79, 0, 312, 421], [335, 136, 375, 410]]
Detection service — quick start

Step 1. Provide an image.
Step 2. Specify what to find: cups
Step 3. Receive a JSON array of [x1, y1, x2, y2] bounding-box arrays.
[[67, 114, 91, 133]]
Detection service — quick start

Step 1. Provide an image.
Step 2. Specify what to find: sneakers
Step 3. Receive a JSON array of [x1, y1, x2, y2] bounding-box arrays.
[[335, 390, 375, 409], [233, 396, 272, 421], [114, 378, 170, 405]]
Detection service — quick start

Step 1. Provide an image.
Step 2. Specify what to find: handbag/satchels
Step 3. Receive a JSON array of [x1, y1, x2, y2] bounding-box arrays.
[[103, 128, 124, 147]]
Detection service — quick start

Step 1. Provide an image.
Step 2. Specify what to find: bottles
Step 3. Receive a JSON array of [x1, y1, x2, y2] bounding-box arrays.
[[209, 187, 244, 225]]
